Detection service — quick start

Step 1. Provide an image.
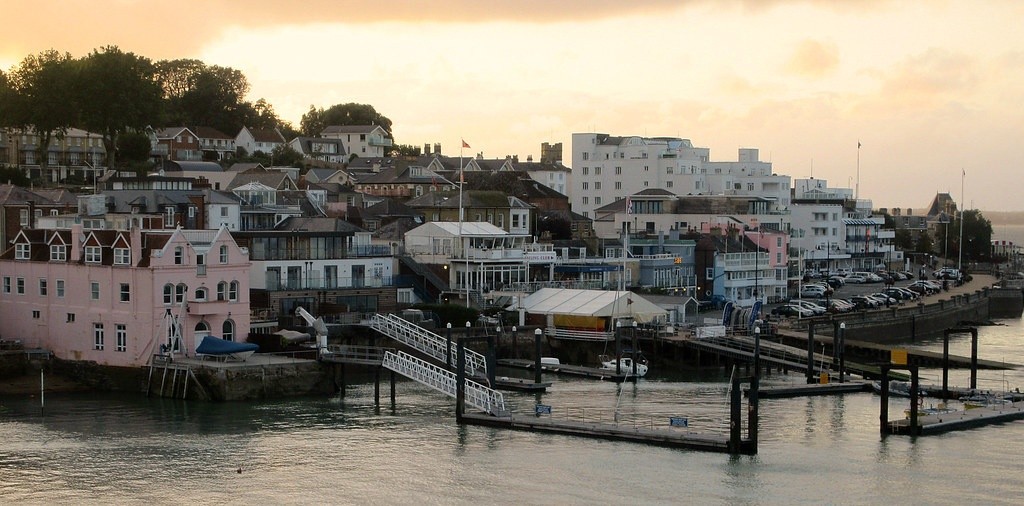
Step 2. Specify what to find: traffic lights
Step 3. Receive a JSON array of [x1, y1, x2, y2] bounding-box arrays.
[[674, 288, 678, 292], [683, 288, 687, 292]]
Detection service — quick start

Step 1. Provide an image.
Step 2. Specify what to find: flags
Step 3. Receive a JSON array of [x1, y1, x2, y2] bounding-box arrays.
[[627, 199, 633, 214], [462, 139, 471, 148]]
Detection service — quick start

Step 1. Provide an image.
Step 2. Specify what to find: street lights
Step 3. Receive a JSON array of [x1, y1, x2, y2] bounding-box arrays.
[[447, 323, 452, 369], [754, 326, 760, 390], [466, 322, 470, 350], [512, 326, 516, 357], [840, 321, 846, 386], [534, 328, 537, 384], [496, 326, 501, 358]]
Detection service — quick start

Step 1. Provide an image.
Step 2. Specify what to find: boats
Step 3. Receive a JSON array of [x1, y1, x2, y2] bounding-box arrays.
[[602, 357, 648, 379], [960, 395, 1011, 411], [903, 405, 956, 419], [867, 382, 928, 398]]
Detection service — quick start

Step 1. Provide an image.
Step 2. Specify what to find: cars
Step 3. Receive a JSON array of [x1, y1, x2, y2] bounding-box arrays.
[[855, 280, 940, 309], [833, 271, 914, 282], [799, 270, 843, 297], [932, 267, 962, 280], [774, 298, 855, 316]]
